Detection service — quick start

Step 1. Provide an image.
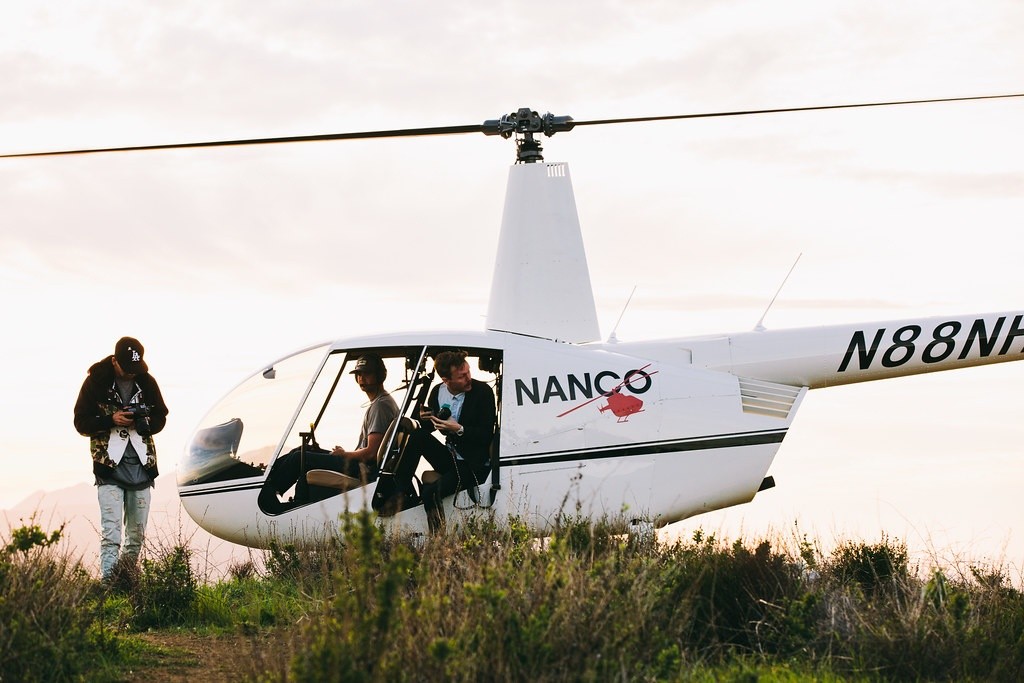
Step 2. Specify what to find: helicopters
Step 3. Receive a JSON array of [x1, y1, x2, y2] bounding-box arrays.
[[0, 94, 1024, 555]]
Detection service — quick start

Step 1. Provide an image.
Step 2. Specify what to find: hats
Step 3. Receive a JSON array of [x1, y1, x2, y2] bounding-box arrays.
[[349, 353, 386, 374], [115, 337, 148, 374]]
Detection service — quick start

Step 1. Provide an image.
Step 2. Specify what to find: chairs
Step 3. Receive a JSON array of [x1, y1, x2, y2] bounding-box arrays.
[[305, 415, 420, 492], [422, 425, 497, 484]]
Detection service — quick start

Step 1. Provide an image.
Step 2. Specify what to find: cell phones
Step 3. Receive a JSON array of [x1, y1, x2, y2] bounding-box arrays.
[[422, 405, 432, 418]]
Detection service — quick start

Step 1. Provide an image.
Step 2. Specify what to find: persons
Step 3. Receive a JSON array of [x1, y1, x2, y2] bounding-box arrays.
[[263, 354, 400, 496], [74, 337, 169, 589], [376, 350, 495, 538]]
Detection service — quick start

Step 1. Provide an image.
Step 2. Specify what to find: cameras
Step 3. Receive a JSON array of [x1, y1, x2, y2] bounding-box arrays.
[[122, 402, 153, 421]]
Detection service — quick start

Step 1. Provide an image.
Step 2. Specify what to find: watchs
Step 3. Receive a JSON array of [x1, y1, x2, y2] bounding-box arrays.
[[455, 426, 464, 437]]
